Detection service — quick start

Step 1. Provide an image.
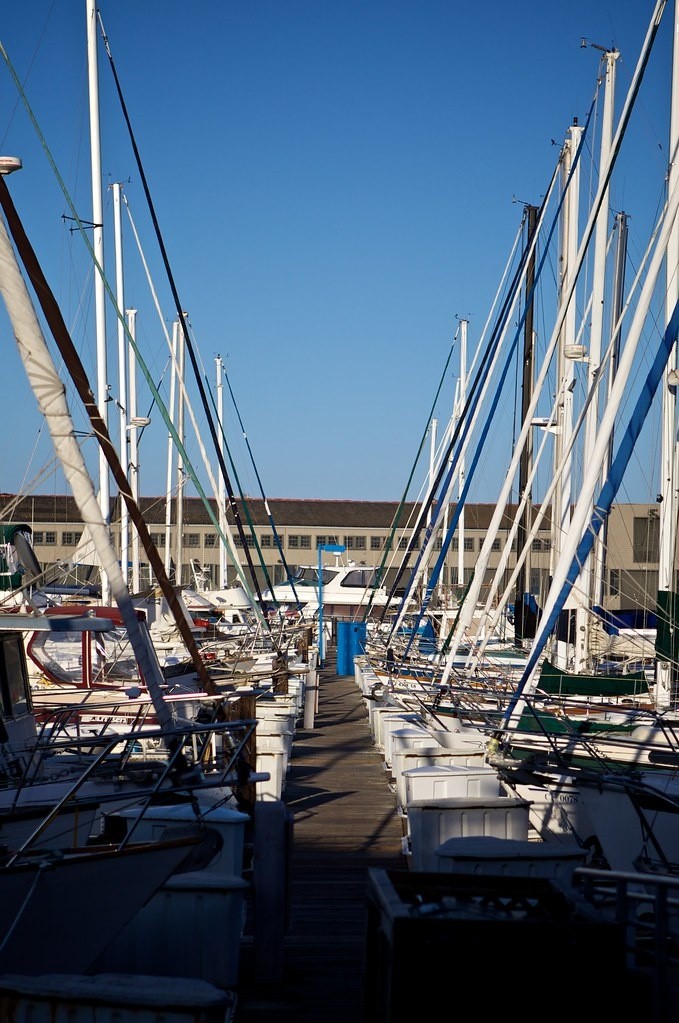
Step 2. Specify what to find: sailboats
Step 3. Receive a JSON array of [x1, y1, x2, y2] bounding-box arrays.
[[1, 0, 679, 1023]]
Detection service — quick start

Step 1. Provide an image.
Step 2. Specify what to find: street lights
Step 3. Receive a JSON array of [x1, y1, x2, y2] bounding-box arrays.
[[318, 542, 347, 670]]
